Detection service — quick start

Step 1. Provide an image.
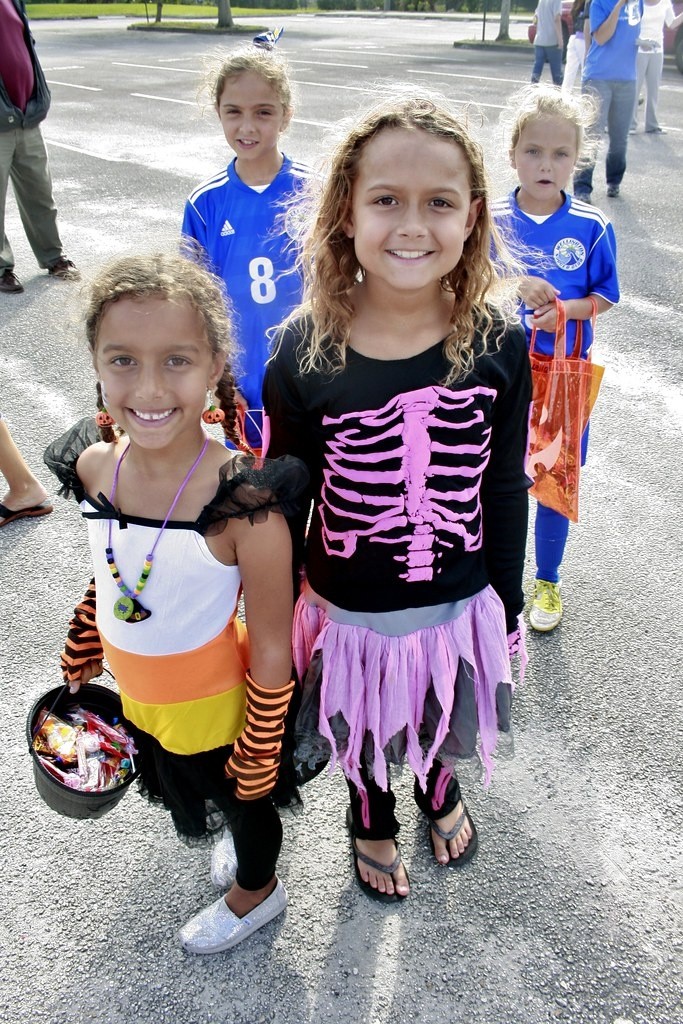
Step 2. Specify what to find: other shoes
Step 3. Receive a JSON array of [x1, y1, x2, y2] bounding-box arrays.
[[178, 875, 288, 954], [0, 272, 24, 294], [606, 185, 618, 197], [210, 828, 239, 890], [630, 128, 637, 134], [48, 257, 80, 277], [646, 127, 667, 134], [575, 194, 592, 204]]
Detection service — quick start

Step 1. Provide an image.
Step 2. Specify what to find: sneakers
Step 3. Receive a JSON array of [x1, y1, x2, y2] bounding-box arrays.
[[524, 578, 563, 630]]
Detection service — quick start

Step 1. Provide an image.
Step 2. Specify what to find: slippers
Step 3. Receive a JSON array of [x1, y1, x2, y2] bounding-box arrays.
[[348, 807, 409, 904], [0, 500, 53, 528], [428, 799, 478, 868]]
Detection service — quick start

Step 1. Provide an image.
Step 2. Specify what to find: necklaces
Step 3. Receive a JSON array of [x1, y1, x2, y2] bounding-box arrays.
[[106, 425, 209, 623]]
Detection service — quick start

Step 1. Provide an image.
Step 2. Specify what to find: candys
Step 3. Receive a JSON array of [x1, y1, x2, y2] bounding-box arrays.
[[30, 708, 138, 793]]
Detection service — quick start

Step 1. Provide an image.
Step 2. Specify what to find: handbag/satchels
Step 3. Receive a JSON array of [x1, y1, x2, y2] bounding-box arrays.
[[526, 294, 606, 524]]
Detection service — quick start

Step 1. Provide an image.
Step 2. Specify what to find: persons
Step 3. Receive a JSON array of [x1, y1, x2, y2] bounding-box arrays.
[[179, 51, 328, 470], [0, 418, 53, 526], [264, 94, 534, 902], [489, 90, 620, 629], [531, 0, 683, 203], [62, 253, 295, 953], [0, 0, 82, 294]]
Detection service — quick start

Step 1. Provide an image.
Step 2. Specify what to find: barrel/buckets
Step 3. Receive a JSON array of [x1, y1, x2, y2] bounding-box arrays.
[[26, 667, 139, 820]]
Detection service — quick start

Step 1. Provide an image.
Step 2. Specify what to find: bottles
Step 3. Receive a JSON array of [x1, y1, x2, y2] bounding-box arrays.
[[627, 0, 641, 26]]
